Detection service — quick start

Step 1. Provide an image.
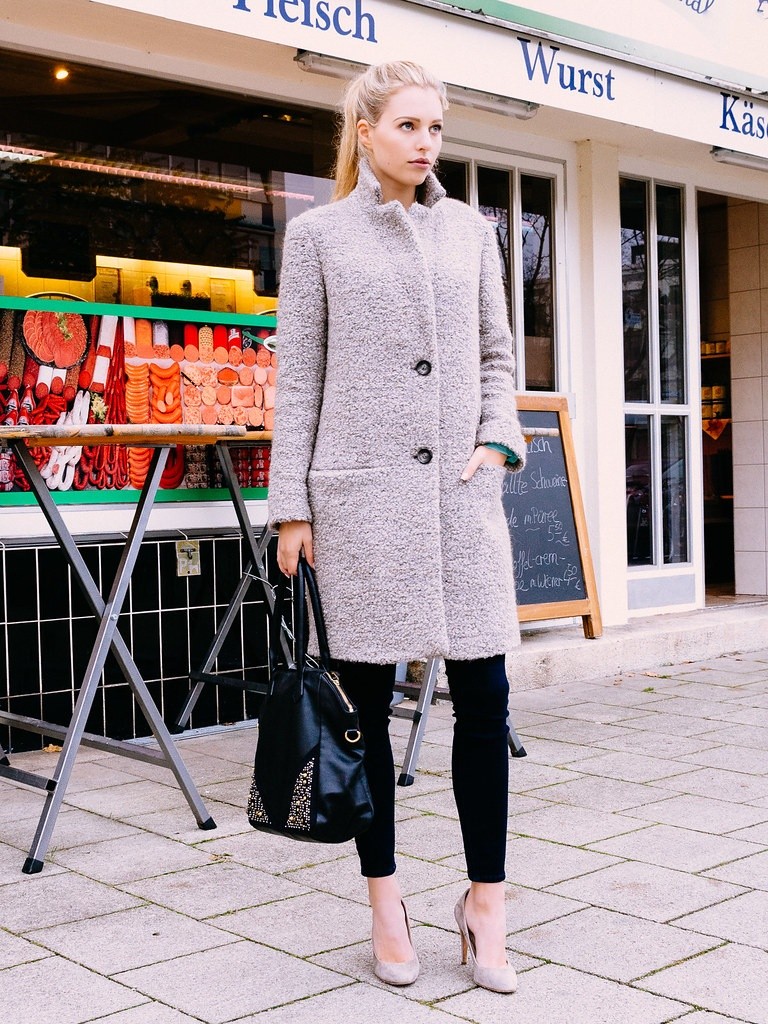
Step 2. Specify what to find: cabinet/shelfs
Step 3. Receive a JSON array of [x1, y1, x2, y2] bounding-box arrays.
[[701, 353, 732, 441]]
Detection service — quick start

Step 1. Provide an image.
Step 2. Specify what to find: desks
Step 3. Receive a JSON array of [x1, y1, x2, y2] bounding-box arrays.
[[172, 430, 536, 788], [0, 424, 247, 874]]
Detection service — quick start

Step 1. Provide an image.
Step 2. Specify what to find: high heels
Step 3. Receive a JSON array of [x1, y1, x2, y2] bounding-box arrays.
[[451, 888, 519, 994], [369, 895, 421, 986]]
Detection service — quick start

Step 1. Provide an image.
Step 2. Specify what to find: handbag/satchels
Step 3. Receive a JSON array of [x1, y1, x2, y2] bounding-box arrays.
[[246, 556, 379, 843]]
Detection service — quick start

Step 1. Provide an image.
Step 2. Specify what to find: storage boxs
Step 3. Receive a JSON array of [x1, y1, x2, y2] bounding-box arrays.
[[525, 336, 552, 387], [6, 131, 262, 181]]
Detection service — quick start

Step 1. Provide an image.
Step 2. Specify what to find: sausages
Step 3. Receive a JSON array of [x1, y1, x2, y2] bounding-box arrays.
[[0, 308, 185, 491]]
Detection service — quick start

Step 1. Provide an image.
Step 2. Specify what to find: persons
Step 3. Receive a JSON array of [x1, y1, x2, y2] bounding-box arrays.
[[268, 57, 525, 994]]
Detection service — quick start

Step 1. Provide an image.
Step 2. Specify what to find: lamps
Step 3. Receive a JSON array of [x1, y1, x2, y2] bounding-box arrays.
[[709, 146, 768, 173], [293, 48, 539, 120]]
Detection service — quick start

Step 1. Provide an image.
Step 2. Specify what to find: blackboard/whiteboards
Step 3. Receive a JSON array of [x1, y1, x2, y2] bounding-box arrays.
[[500, 394, 597, 623]]
